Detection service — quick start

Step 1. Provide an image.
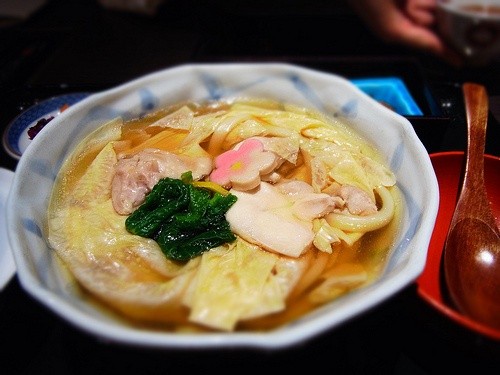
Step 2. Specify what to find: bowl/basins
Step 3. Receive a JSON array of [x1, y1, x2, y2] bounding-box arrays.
[[417, 151, 500, 339]]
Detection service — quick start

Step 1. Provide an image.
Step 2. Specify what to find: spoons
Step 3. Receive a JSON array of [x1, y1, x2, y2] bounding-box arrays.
[[443, 83, 500, 330]]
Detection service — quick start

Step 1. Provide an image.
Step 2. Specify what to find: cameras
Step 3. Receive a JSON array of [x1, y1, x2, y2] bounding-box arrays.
[[333, 61, 451, 153]]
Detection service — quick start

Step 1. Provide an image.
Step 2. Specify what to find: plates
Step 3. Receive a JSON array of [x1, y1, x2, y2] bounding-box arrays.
[[6, 62, 441, 353], [2, 92, 94, 160]]
